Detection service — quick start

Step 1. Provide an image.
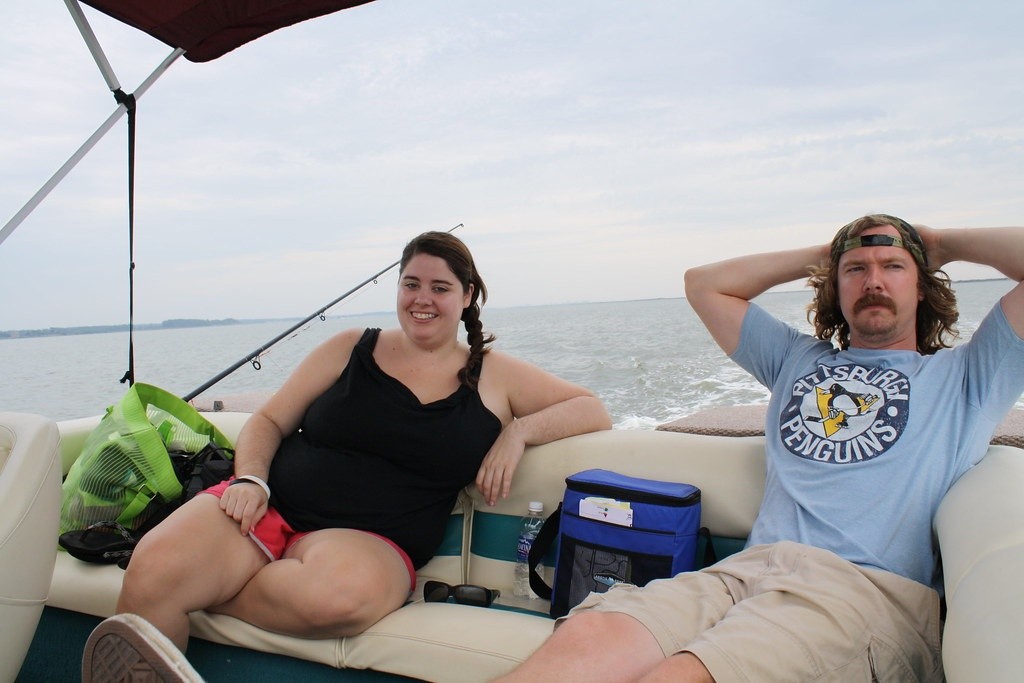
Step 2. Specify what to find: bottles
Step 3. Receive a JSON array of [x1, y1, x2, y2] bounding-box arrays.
[[513, 501, 544, 601]]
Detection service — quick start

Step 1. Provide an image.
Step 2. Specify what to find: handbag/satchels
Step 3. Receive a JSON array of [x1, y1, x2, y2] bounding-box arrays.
[[54, 382, 237, 553], [527, 469, 719, 622]]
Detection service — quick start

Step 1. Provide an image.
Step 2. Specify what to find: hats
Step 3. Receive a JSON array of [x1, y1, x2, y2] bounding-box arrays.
[[829, 214, 930, 269]]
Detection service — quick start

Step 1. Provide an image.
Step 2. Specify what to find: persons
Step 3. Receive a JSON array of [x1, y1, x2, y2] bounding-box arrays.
[[79, 212, 1024, 683], [109, 228, 616, 666]]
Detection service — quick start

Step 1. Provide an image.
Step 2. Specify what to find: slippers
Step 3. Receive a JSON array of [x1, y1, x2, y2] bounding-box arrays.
[[58, 520, 144, 570]]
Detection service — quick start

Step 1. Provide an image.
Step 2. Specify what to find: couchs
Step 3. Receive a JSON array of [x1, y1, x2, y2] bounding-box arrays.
[[46, 409, 1022, 683]]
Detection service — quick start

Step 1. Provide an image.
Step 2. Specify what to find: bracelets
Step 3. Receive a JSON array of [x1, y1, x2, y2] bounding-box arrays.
[[227, 474, 271, 501]]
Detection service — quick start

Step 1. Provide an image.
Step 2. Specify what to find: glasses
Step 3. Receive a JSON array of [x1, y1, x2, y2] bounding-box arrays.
[[423, 580, 501, 609]]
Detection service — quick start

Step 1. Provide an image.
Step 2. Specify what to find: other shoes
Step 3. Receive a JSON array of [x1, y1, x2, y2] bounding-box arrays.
[[81, 611, 206, 683]]
